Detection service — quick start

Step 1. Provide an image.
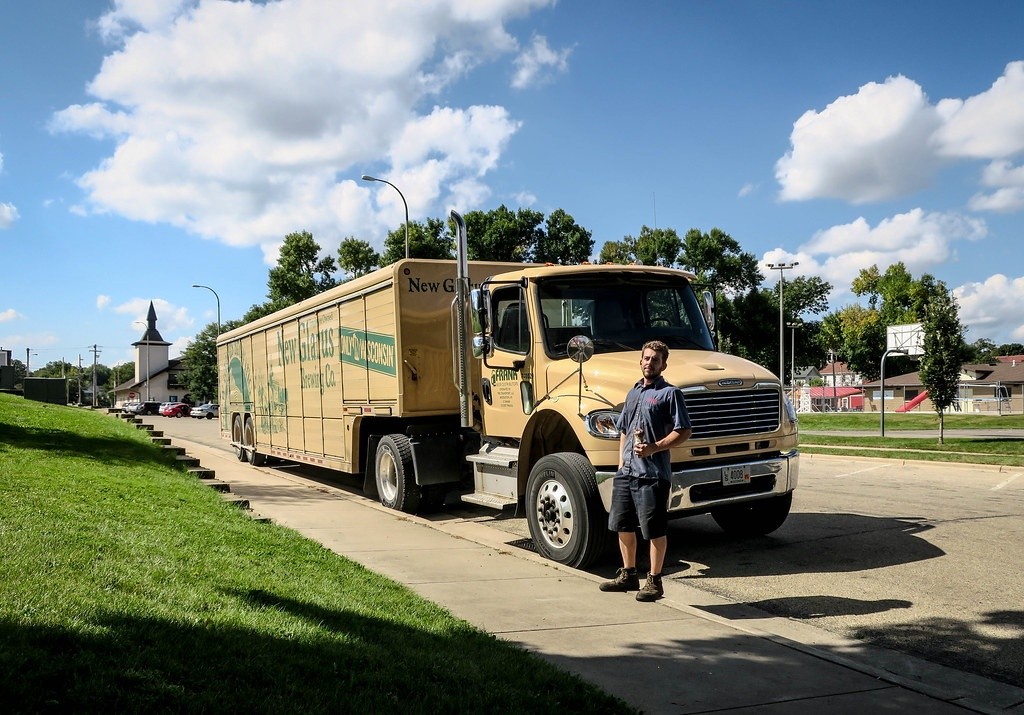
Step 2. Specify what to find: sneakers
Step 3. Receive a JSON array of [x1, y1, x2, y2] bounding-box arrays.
[[599, 568, 640, 591], [636, 572, 663, 602]]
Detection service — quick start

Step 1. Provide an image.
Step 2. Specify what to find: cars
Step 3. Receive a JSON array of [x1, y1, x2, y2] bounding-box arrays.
[[122, 401, 192, 418], [189, 403, 219, 419]]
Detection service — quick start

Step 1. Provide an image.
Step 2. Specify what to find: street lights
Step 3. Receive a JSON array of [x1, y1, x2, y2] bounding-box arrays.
[[134, 321, 150, 401], [765, 261, 800, 406], [786, 321, 803, 407], [362, 174, 409, 259], [192, 284, 220, 336]]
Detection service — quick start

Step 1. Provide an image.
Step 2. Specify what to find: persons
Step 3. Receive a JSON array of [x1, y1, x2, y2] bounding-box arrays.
[[599, 341, 692, 603]]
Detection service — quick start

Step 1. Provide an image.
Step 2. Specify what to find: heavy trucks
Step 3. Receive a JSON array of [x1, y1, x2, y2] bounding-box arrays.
[[216, 206, 804, 574]]
